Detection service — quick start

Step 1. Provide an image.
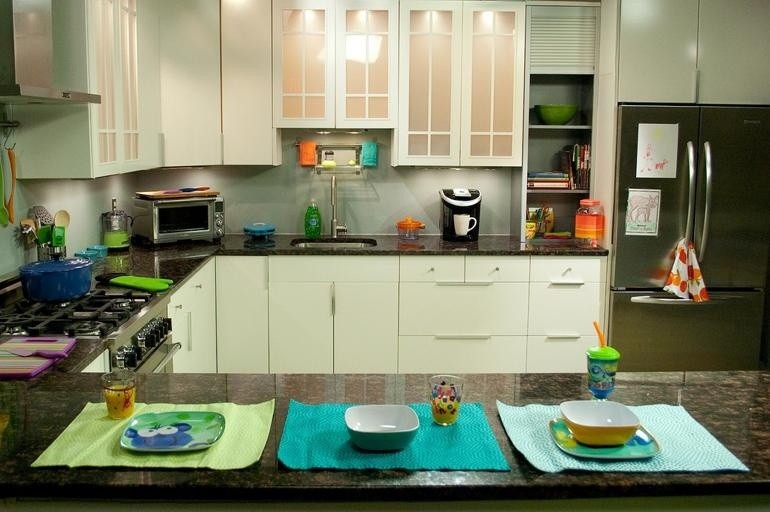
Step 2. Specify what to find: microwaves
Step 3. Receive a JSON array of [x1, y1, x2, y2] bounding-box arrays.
[[130, 196, 225, 246]]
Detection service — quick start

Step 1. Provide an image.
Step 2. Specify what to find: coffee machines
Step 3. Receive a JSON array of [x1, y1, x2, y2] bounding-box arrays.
[[439, 188, 482, 242]]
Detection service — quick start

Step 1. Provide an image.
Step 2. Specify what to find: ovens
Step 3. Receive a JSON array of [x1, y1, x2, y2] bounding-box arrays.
[[136, 336, 182, 374]]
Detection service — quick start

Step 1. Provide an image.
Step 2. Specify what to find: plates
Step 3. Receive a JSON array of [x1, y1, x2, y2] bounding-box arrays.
[[121, 411, 225, 451], [548, 417, 661, 460]]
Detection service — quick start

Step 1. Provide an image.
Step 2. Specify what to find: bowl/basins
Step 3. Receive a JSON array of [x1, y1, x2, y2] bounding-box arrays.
[[534, 104, 578, 124], [346, 404, 420, 453], [560, 400, 642, 444]]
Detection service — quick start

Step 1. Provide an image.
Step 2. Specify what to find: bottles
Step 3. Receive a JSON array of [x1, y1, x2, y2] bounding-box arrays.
[[575, 199, 605, 249], [325, 151, 335, 162]]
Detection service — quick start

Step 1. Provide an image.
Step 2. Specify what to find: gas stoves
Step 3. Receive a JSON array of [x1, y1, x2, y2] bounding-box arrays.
[[0, 288, 172, 374]]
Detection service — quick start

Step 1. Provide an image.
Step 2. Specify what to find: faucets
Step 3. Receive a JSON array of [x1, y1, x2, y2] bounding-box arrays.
[[330, 176, 347, 238]]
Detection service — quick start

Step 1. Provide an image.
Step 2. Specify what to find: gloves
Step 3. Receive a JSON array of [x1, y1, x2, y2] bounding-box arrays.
[[95, 273, 173, 291]]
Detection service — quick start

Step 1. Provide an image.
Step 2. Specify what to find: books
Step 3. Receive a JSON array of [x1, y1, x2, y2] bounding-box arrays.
[[528, 141, 592, 190]]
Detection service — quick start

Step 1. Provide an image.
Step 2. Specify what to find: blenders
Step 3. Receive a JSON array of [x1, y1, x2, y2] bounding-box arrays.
[[100, 208, 134, 252]]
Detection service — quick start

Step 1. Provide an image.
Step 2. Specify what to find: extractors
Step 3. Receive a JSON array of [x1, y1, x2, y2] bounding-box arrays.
[[1, 1, 101, 107]]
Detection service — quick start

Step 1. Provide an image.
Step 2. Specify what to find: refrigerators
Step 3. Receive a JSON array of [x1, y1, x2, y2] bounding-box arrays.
[[606, 105, 769, 371]]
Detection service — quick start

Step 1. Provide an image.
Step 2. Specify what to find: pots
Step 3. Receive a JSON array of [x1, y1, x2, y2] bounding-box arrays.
[[18, 253, 94, 301]]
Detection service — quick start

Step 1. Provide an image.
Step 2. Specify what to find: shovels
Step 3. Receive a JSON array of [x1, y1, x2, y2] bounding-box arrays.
[[20, 218, 36, 232]]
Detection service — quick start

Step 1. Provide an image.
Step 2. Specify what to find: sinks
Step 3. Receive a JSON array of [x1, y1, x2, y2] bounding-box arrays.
[[290, 237, 378, 248]]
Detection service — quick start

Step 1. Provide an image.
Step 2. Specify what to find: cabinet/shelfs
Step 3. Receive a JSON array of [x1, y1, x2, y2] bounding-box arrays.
[[617, 0, 770, 107], [510, 2, 601, 243], [167, 258, 216, 375]]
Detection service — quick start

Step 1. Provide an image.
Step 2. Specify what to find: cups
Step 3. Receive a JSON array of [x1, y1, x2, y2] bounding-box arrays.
[[102, 370, 136, 419], [527, 220, 537, 240], [429, 374, 467, 427], [75, 251, 97, 263], [586, 345, 622, 399], [454, 215, 478, 236], [87, 245, 107, 258]]
